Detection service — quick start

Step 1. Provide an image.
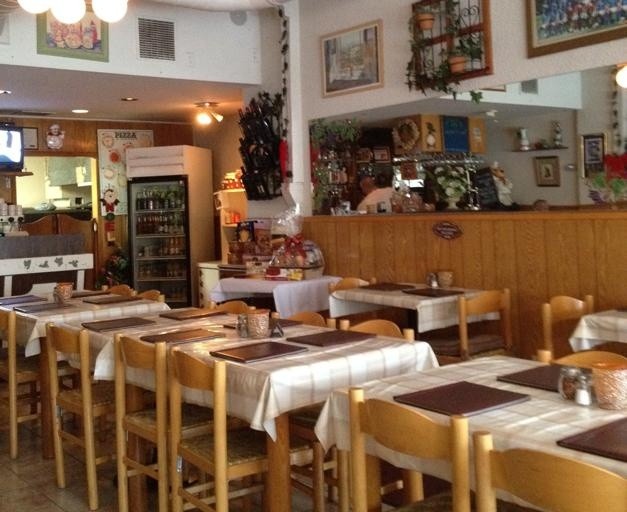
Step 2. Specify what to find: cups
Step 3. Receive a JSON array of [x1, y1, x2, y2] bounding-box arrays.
[[57, 281, 75, 306], [376, 201, 387, 213], [247, 308, 270, 338], [340, 201, 350, 217], [593, 361, 627, 411], [0, 196, 23, 215], [367, 205, 377, 216]]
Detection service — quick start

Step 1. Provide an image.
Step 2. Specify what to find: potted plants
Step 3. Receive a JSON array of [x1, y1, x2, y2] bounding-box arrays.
[[401, 0, 462, 51], [433, 40, 482, 105]]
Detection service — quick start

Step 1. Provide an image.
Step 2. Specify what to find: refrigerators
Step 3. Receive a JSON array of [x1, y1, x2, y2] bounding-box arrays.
[[124, 144, 217, 308]]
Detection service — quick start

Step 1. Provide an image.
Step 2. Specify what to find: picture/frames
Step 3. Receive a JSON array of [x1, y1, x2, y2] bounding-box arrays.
[[522, 0, 627, 60], [533, 155, 561, 189], [478, 85, 507, 94], [578, 130, 608, 180], [33, 4, 110, 65], [317, 17, 384, 100]]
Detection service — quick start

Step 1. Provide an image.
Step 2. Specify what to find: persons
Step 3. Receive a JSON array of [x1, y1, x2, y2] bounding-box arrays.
[[355, 171, 397, 211]]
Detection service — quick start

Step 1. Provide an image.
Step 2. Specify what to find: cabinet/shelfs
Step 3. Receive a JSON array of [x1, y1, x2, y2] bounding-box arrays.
[[216, 190, 250, 262], [388, 110, 486, 155], [239, 106, 284, 202]]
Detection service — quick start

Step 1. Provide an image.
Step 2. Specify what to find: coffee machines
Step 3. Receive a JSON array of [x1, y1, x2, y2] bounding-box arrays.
[[0, 215, 30, 237]]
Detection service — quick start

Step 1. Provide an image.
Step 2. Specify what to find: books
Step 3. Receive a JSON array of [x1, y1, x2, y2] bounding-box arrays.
[[81, 317, 156, 332], [0, 295, 48, 305], [82, 295, 143, 304], [360, 280, 415, 292], [209, 340, 308, 364], [497, 364, 592, 393], [392, 380, 530, 417], [223, 318, 303, 328], [159, 309, 228, 321], [72, 291, 111, 299], [404, 288, 465, 298], [140, 328, 226, 345], [285, 328, 377, 346], [13, 303, 73, 313], [557, 415, 627, 461]]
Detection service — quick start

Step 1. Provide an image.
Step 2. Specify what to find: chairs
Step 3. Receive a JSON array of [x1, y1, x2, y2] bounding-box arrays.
[[1, 272, 431, 512], [349, 288, 627, 512]]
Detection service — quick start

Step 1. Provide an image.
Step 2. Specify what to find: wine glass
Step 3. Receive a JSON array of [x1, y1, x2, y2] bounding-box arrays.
[[399, 153, 486, 169]]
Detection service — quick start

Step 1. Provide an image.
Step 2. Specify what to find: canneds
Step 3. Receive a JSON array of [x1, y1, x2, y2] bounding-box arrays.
[[558, 367, 582, 397]]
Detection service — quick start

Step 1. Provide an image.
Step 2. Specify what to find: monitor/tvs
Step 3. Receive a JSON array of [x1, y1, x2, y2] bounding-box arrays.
[[0, 126, 23, 171]]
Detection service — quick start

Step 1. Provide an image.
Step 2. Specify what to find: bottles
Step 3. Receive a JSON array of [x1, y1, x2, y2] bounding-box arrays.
[[237, 107, 280, 199], [238, 306, 256, 338], [136, 187, 186, 300], [269, 312, 284, 338], [320, 144, 349, 184], [559, 365, 592, 406], [45, 287, 59, 303]]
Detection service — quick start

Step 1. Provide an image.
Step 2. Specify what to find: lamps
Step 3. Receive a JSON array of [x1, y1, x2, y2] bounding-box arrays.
[[195, 102, 227, 125]]
